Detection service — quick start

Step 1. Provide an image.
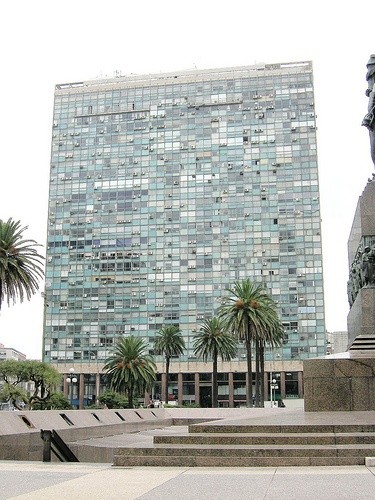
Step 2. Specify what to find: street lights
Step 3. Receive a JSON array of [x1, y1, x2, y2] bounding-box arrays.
[[66, 368, 78, 408], [269, 377, 279, 407]]
